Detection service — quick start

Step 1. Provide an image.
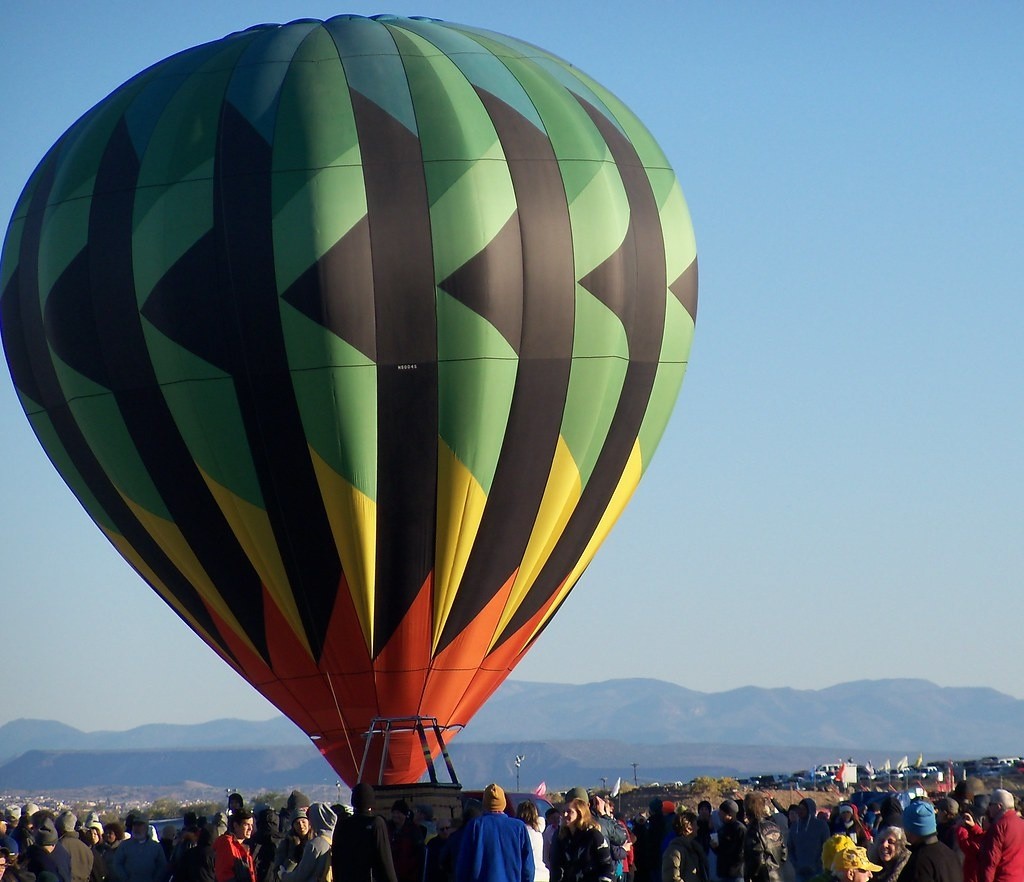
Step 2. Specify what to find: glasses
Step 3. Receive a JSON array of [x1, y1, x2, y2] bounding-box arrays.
[[436, 827, 452, 831], [989, 802, 999, 806]]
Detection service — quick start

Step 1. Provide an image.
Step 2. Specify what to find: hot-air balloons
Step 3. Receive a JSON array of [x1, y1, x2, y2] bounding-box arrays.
[[1, 13, 698, 821]]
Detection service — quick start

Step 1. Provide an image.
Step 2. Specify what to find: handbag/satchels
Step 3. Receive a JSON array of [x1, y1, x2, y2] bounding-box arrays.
[[610, 844, 627, 860]]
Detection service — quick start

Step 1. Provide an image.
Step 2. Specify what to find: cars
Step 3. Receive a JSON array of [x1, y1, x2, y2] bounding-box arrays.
[[919, 766, 937, 773], [782, 778, 807, 790], [738, 779, 753, 789], [998, 758, 1020, 766], [148, 817, 186, 841], [815, 765, 841, 776], [889, 768, 910, 781], [460, 791, 554, 817]]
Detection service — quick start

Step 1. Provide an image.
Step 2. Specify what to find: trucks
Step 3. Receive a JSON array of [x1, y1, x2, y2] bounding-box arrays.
[[845, 765, 877, 784]]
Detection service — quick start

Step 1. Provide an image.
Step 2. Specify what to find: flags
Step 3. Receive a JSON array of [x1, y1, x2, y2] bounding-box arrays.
[[865, 761, 871, 775], [805, 767, 815, 783], [896, 755, 909, 773], [915, 754, 923, 768], [884, 761, 891, 774], [610, 778, 620, 798], [834, 763, 846, 781], [534, 782, 547, 796]]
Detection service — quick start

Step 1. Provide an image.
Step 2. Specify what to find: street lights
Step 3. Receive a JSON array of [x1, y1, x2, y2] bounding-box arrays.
[[514, 755, 525, 792], [600, 777, 607, 789], [630, 763, 639, 787]]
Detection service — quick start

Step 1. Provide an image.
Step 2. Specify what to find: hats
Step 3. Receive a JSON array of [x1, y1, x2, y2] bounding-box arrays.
[[5, 805, 21, 821], [565, 788, 589, 804], [21, 801, 39, 816], [31, 807, 58, 826], [483, 784, 507, 811], [615, 798, 959, 872], [183, 812, 197, 827], [416, 804, 434, 817], [287, 790, 310, 809], [125, 809, 141, 827], [351, 783, 377, 808], [133, 814, 149, 824], [290, 808, 308, 825], [391, 800, 409, 816], [35, 818, 58, 846], [0, 837, 18, 855]]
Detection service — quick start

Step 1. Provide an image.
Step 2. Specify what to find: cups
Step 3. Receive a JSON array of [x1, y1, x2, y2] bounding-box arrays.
[[710, 832, 719, 846], [284, 859, 297, 873]]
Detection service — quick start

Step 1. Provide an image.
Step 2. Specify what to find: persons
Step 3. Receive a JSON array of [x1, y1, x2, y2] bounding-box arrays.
[[0, 780, 1024, 882]]
